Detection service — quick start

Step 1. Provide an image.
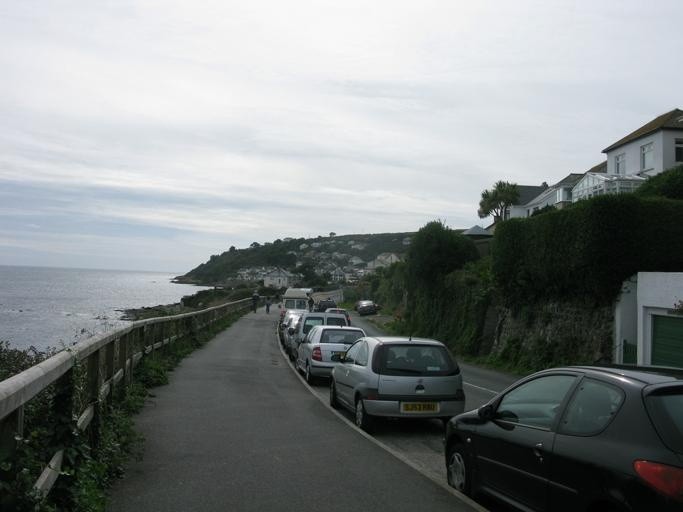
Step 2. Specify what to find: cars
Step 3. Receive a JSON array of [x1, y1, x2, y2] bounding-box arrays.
[[355, 299, 381, 316], [330, 337, 465, 428], [297, 325, 368, 383], [445, 364, 683, 512], [280, 288, 351, 361]]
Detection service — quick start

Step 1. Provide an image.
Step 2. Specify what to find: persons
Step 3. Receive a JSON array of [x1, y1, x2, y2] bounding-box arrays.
[[307, 296, 314, 312], [251, 289, 282, 315]]
